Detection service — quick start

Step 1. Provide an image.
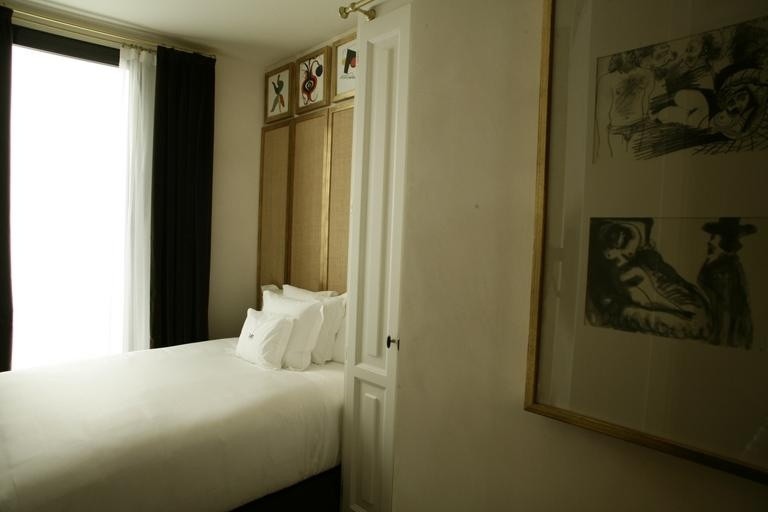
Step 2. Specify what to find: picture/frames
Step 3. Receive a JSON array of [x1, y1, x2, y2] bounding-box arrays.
[[522, 0, 768, 486], [263, 31, 357, 124]]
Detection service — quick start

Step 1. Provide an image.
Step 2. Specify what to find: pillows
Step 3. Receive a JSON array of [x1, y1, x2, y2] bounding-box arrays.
[[260, 283, 338, 302], [282, 285, 348, 365], [333, 304, 349, 366], [260, 290, 325, 371], [236, 307, 295, 373]]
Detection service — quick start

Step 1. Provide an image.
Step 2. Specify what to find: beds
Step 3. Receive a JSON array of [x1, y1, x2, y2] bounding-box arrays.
[[0, 330, 357, 512]]
